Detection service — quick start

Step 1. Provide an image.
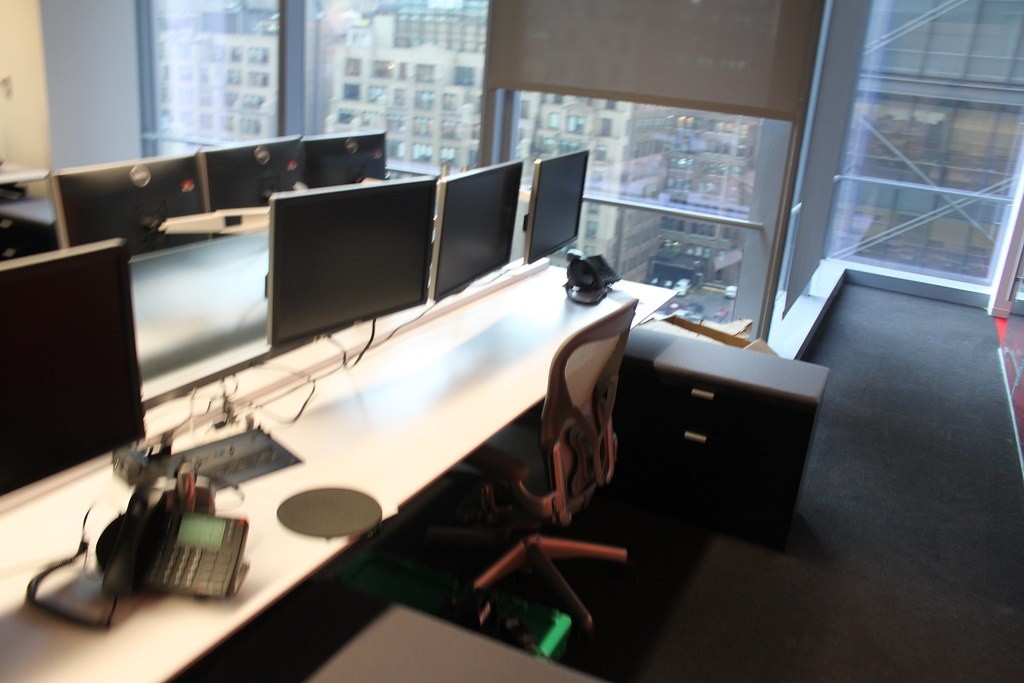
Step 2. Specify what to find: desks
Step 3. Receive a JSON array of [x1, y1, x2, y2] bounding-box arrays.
[[0, 197, 676, 682]]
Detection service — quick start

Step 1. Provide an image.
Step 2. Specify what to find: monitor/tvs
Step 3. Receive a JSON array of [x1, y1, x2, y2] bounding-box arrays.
[[0, 128, 588, 499]]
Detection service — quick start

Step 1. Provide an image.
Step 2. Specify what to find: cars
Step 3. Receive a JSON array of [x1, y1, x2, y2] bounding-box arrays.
[[644, 276, 737, 322]]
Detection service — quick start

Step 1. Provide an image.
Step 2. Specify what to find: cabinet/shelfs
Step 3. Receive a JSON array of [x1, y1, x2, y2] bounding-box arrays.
[[619, 329, 830, 551]]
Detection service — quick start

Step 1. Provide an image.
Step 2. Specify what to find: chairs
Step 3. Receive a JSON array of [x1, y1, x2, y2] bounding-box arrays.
[[422, 295, 638, 643]]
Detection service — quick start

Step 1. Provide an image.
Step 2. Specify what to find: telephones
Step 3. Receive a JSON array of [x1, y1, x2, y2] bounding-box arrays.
[[565, 248, 622, 289], [96, 506, 249, 601]]
